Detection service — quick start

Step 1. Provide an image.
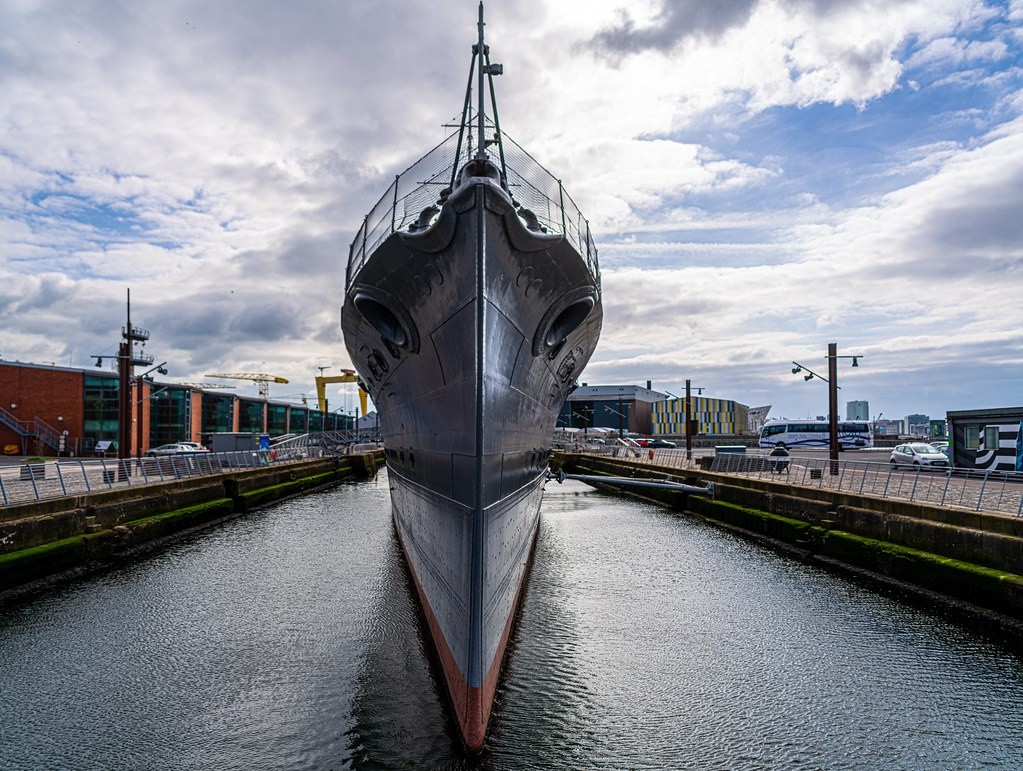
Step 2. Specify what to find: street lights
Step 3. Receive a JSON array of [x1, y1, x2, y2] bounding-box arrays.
[[90, 353, 167, 478], [791, 342, 864, 475], [682, 379, 706, 460]]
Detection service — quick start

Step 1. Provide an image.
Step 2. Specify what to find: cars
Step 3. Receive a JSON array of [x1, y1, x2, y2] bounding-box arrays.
[[902, 441, 949, 457], [554, 426, 676, 448], [146, 444, 195, 456], [890, 443, 949, 472]]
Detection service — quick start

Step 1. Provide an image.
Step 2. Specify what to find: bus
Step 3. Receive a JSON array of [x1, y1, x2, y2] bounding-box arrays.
[[758, 420, 874, 452]]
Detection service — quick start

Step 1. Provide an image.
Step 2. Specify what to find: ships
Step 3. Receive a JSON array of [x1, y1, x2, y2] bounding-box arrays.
[[341, 1, 605, 754]]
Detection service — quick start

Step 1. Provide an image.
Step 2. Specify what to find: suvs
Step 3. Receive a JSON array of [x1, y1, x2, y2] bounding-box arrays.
[[175, 441, 204, 451]]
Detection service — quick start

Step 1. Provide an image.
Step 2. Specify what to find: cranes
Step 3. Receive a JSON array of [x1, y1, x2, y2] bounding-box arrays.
[[179, 382, 237, 391], [205, 372, 291, 401], [268, 392, 317, 405]]
